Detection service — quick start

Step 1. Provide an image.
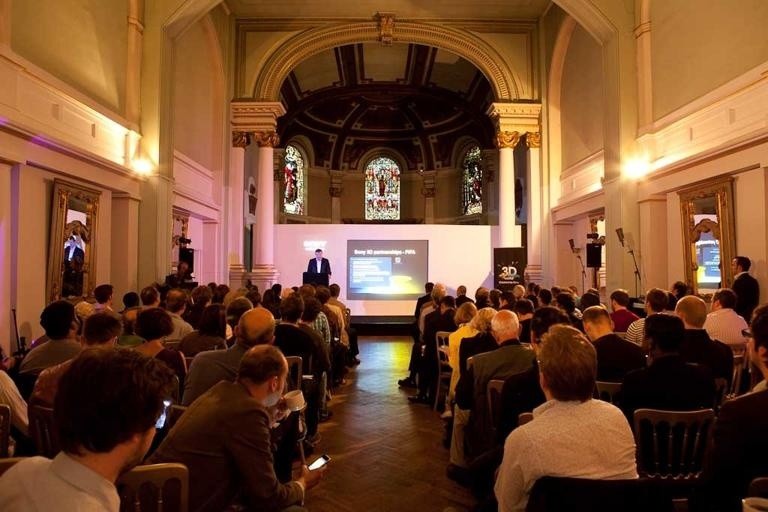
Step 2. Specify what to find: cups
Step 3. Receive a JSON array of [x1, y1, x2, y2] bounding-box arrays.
[[284, 389, 308, 411], [741, 497, 768, 512]]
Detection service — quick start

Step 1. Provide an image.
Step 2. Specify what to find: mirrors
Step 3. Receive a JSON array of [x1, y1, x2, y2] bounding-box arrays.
[[169, 206, 190, 276], [675, 176, 738, 300], [588, 212, 608, 302], [46, 178, 104, 305]]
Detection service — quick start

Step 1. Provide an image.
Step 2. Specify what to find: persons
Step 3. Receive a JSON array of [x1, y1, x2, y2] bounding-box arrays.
[[64, 237, 84, 262], [308, 249, 331, 279], [732, 255, 760, 324], [1, 264, 361, 511], [399, 281, 768, 512]]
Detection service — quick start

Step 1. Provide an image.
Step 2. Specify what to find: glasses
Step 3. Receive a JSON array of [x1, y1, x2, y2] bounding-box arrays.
[[741, 327, 762, 340]]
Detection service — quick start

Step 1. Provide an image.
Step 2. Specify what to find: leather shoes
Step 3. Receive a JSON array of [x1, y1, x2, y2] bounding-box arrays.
[[398, 378, 411, 388], [407, 393, 427, 402]]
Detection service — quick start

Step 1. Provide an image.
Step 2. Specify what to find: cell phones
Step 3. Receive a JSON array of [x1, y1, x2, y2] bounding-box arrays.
[[307, 454, 332, 471], [155, 399, 173, 430]]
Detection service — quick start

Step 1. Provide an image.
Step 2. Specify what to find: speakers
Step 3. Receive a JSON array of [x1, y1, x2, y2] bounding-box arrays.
[[179, 248, 194, 273], [586, 244, 601, 268]]
